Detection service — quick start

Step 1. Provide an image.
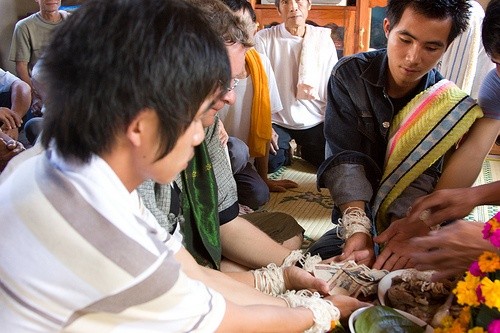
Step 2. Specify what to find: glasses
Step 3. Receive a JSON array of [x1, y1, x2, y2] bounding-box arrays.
[[216, 79, 240, 95]]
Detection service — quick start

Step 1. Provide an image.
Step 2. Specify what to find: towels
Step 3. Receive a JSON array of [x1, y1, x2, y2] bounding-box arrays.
[[372, 79, 483, 260], [434, 0, 485, 96], [244, 46, 273, 161], [179, 138, 221, 270], [296, 24, 332, 101]]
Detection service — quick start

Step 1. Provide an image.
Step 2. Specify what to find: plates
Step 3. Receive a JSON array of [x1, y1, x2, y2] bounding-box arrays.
[[348, 305, 434, 333], [378, 268, 450, 307]]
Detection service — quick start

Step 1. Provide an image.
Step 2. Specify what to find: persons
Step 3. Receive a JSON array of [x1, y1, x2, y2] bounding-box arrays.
[[1, 2, 500, 333]]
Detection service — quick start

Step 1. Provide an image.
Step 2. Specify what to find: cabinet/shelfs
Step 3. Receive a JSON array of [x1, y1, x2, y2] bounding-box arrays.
[[250, 0, 391, 60]]
